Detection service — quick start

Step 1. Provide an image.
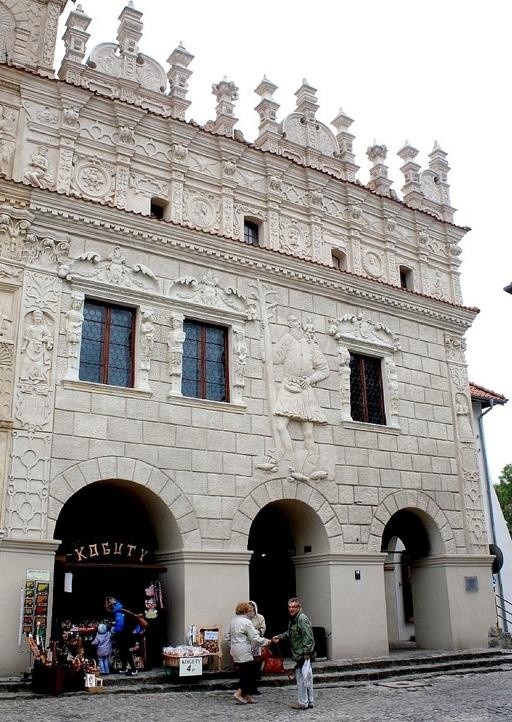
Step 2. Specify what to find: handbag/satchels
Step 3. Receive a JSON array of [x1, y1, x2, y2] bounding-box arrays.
[[135, 613, 149, 629], [143, 577, 166, 619]]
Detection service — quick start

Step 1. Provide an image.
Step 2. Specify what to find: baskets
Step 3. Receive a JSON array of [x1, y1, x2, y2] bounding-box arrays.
[[86, 658, 101, 676]]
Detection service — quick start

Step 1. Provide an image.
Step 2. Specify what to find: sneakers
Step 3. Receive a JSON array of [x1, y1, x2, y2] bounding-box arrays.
[[291, 701, 314, 709], [232, 690, 261, 704]]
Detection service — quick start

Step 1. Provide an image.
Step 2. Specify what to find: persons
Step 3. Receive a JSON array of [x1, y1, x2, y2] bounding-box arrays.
[[258, 314, 332, 474], [247, 600, 267, 695], [104, 596, 146, 676], [224, 602, 279, 704], [275, 597, 318, 710], [23, 145, 49, 190], [66, 297, 85, 358], [90, 624, 112, 675], [20, 310, 53, 382]]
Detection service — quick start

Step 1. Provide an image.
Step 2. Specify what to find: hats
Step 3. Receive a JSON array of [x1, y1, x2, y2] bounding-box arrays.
[[98, 623, 107, 633]]
